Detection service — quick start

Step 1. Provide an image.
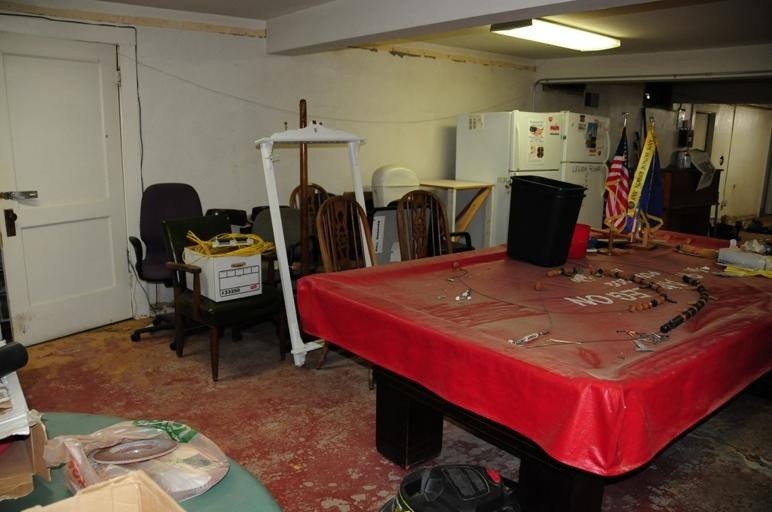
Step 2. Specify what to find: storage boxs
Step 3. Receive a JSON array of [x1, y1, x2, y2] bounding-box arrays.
[[0, 339, 51, 503], [184, 243, 264, 305], [21, 469, 184, 512]]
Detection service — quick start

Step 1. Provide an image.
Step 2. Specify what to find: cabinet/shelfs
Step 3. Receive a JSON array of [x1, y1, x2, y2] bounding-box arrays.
[[659, 168, 725, 235], [420, 179, 495, 247]]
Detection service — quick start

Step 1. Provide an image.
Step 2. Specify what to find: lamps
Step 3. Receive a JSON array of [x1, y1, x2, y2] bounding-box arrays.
[[489, 16, 622, 52]]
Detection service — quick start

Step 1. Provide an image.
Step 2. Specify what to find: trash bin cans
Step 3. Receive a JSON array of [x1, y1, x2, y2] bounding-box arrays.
[[371, 165, 420, 207], [508, 175, 588, 268]]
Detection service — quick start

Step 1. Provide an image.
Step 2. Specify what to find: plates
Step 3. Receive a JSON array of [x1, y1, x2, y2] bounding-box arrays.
[[94, 436, 181, 468], [59, 418, 231, 506]]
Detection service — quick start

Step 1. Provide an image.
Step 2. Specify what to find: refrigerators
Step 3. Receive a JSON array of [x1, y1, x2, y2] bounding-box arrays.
[[455, 111, 564, 252], [558, 112, 610, 237]]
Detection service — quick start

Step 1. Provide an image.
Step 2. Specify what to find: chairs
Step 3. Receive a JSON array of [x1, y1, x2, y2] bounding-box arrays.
[[315, 194, 378, 390], [246, 206, 302, 287], [127, 181, 205, 350], [161, 215, 286, 381], [395, 187, 452, 261], [289, 185, 328, 274]]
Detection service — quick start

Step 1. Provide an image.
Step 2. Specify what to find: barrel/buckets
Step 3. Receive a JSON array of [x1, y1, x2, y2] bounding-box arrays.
[[568, 224, 590, 259]]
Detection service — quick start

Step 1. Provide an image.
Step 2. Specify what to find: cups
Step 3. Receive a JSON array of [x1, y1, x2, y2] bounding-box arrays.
[[569, 225, 591, 258]]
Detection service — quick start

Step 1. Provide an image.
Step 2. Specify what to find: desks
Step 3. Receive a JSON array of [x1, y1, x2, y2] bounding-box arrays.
[[295, 227, 772, 512], [0, 409, 280, 511]]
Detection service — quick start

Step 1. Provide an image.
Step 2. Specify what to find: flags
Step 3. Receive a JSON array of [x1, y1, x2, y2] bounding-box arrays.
[[601, 126, 632, 235], [628, 124, 665, 234]]
